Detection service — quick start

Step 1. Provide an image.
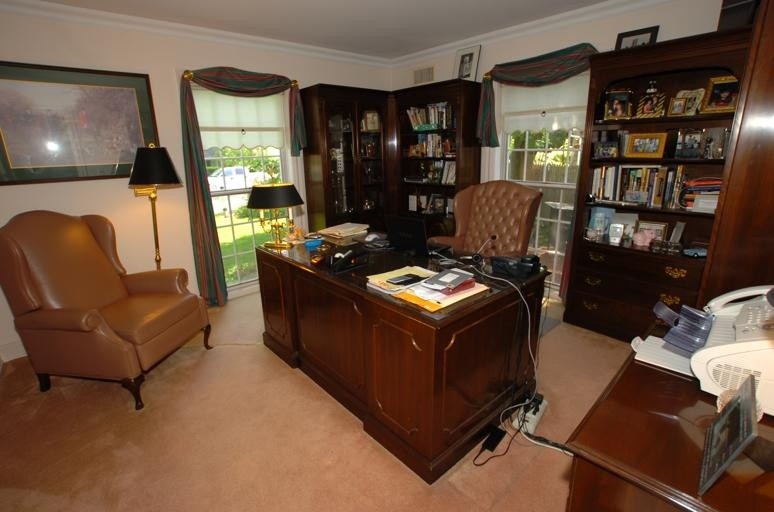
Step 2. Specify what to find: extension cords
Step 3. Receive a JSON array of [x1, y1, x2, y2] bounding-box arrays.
[[512, 394, 547, 434]]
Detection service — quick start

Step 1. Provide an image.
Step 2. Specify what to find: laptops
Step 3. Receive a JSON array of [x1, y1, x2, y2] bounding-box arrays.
[[383, 213, 452, 258]]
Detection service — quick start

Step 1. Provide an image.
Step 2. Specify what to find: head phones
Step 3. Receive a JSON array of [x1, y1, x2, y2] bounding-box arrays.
[[459, 253, 482, 263]]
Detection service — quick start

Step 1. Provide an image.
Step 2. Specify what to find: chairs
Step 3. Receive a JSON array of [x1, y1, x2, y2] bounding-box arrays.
[[0, 207, 213, 410], [426, 178, 543, 256]]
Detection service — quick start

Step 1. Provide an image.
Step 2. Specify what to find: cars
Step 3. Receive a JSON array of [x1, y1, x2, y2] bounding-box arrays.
[[207, 166, 274, 192]]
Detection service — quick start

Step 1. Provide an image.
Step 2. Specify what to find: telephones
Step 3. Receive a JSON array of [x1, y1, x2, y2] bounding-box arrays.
[[705, 284, 774, 316], [517, 254, 539, 273]]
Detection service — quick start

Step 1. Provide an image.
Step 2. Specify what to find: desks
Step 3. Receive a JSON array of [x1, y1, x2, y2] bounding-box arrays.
[[560, 323, 774, 511], [252, 227, 552, 486]]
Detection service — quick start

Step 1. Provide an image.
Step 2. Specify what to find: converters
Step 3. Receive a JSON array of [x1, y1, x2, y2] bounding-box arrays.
[[531, 393, 543, 404]]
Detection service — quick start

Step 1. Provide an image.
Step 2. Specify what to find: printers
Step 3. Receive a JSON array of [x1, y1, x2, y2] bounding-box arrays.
[[629, 284, 774, 422]]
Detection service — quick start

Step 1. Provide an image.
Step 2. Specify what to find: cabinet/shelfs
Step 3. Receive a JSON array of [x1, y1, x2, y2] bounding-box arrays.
[[297, 80, 389, 234], [695, 1, 774, 312], [558, 28, 752, 344], [389, 78, 484, 239]]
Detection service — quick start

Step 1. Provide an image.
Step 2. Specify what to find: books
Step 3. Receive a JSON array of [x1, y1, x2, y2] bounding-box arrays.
[[591, 165, 721, 215], [405, 101, 456, 185], [366, 264, 489, 314]]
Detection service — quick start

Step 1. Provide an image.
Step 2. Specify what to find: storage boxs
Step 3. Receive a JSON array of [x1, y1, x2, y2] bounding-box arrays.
[[715, 0, 758, 33]]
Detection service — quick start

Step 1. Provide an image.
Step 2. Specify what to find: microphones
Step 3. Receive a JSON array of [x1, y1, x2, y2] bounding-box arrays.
[[476, 235, 496, 254]]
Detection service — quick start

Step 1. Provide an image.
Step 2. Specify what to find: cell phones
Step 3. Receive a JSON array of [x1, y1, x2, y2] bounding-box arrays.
[[482, 428, 506, 452], [386, 273, 419, 285]]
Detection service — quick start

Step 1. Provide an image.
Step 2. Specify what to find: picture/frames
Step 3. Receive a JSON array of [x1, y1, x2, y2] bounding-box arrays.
[[0, 57, 160, 188], [622, 132, 669, 159], [666, 96, 687, 115], [426, 193, 447, 217], [451, 44, 484, 82], [363, 110, 380, 133], [612, 24, 660, 51], [667, 87, 706, 117], [699, 74, 737, 114], [635, 219, 669, 244], [694, 373, 760, 500], [438, 159, 456, 188], [634, 92, 666, 119], [602, 87, 635, 118]]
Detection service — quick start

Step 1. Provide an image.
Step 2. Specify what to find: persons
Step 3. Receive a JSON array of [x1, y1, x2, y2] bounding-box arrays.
[[673, 101, 681, 111], [610, 99, 627, 117], [688, 95, 696, 111], [643, 96, 658, 113], [633, 139, 658, 152], [594, 144, 614, 158], [710, 88, 732, 108], [688, 134, 697, 148], [703, 137, 713, 158]]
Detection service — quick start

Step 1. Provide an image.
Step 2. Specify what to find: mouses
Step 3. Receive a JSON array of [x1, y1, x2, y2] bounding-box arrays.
[[365, 233, 381, 242]]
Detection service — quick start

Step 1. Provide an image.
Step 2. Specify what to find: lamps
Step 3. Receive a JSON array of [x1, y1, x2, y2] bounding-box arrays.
[[126, 142, 184, 272], [247, 182, 306, 251]]
